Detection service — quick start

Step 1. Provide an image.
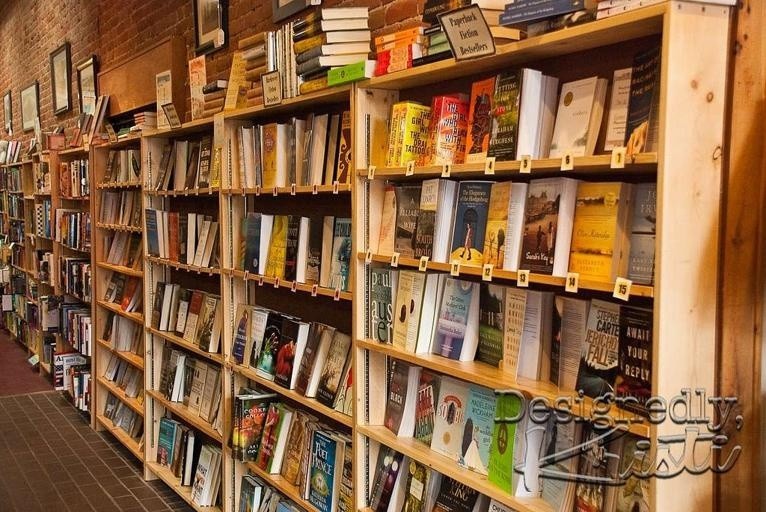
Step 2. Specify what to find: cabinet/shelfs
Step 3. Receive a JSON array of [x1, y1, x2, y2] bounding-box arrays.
[[1, 0, 736, 512]]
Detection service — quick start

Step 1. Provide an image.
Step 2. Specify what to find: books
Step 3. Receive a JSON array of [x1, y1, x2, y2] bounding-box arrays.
[[0, 0, 662, 512]]
